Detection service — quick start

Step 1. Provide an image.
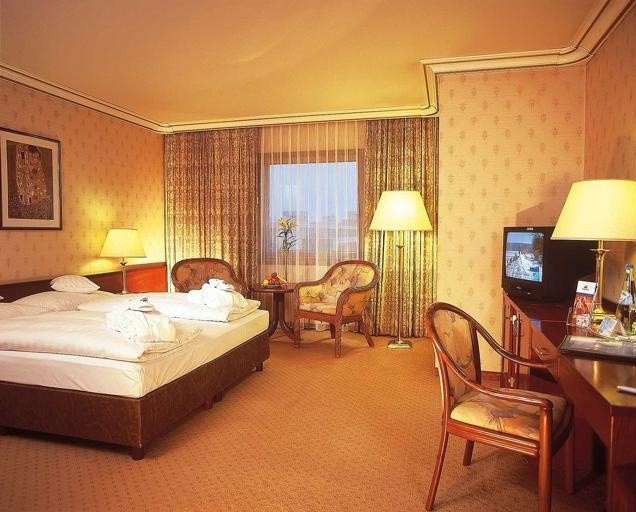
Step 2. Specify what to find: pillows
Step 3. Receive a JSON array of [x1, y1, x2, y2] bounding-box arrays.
[[1, 274, 117, 322]]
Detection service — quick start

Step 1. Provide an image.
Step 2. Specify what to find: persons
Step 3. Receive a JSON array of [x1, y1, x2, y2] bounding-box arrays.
[[581, 297, 589, 315], [572, 299, 583, 326]]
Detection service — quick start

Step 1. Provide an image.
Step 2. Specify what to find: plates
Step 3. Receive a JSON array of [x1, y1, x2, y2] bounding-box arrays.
[[259, 285, 280, 289], [589, 324, 635, 342]]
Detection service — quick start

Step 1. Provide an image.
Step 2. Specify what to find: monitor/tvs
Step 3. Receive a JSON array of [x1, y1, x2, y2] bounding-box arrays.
[[502, 226, 598, 301]]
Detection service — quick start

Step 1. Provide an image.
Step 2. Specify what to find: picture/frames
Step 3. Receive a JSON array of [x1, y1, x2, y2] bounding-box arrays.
[[0, 126, 63, 231]]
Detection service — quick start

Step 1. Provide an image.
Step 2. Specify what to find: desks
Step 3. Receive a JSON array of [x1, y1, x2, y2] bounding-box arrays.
[[502, 290, 636, 510], [254, 282, 304, 344]]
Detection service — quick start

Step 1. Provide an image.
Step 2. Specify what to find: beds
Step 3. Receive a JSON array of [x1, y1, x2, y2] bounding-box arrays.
[[1, 289, 270, 459]]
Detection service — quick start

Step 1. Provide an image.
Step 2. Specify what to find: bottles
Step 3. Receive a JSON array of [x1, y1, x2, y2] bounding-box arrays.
[[615, 264, 636, 335]]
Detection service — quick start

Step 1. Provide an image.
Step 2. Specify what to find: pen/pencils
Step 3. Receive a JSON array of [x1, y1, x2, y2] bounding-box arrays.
[[616, 385, 635, 394]]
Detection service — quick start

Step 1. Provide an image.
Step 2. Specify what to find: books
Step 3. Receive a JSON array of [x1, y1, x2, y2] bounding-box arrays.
[[557, 334, 636, 363], [571, 280, 600, 328]]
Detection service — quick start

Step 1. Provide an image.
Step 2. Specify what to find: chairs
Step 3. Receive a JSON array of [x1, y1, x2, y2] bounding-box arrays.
[[423, 301, 579, 512], [293, 260, 380, 358], [171, 258, 249, 298]]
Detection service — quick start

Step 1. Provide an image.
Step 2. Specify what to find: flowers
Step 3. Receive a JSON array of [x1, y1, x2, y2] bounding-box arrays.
[[273, 216, 299, 280]]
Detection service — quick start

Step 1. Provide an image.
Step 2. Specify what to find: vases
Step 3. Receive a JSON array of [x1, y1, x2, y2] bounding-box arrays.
[[282, 250, 291, 282]]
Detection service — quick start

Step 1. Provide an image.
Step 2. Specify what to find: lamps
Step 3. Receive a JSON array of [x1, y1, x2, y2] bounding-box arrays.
[[550, 176, 636, 322], [369, 189, 434, 349], [98, 228, 147, 295]]
